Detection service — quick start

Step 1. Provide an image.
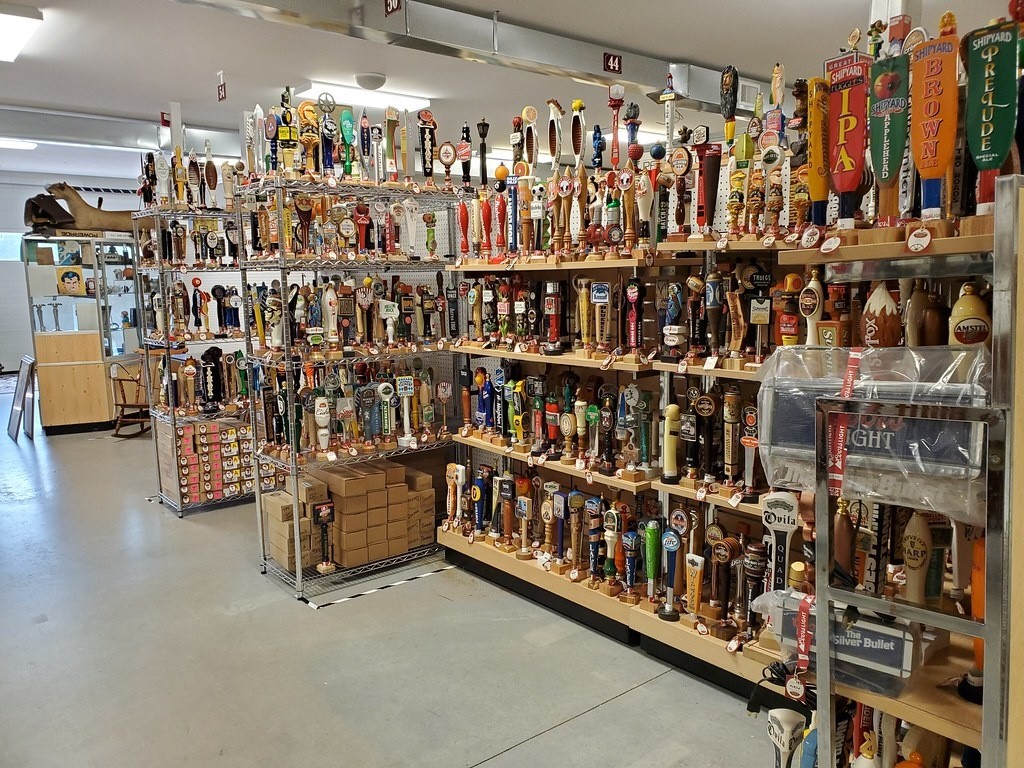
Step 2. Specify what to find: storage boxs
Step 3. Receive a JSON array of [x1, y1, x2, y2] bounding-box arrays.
[[152, 414, 435, 569]]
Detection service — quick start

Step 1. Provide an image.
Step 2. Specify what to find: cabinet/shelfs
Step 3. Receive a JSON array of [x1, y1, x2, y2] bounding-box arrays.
[[124, 204, 253, 517], [229, 157, 466, 599], [437, 185, 1023, 768], [21, 234, 161, 430]]
